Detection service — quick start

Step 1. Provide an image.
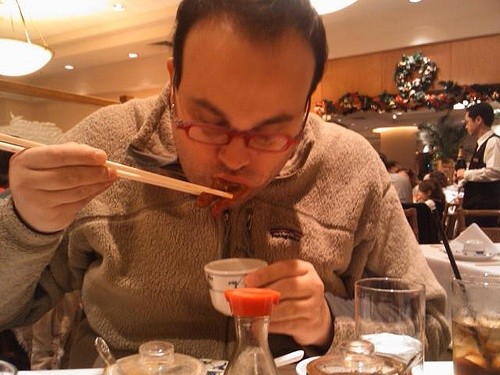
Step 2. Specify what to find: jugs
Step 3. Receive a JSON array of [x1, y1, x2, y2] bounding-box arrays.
[[219, 288, 281, 375]]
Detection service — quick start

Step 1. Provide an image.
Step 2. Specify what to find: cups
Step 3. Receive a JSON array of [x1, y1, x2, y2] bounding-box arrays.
[[450, 272, 500, 375], [354, 278, 426, 375], [463, 240, 486, 256], [0, 360, 18, 375], [204, 258, 268, 317]]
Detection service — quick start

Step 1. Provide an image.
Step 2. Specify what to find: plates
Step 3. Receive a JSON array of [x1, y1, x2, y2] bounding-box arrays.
[[295, 356, 322, 375], [451, 250, 495, 261]]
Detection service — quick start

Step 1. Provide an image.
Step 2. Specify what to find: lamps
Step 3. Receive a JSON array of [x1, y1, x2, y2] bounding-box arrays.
[[0, 0, 57, 77]]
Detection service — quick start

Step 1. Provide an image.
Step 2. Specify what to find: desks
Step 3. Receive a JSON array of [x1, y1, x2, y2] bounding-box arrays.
[[0, 242, 500, 375]]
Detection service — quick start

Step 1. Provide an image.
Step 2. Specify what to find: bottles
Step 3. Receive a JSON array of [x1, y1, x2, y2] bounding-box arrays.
[[107, 340, 207, 375], [455, 144, 467, 184], [306, 340, 411, 375]]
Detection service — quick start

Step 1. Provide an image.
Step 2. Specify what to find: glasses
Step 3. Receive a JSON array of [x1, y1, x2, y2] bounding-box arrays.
[[170, 78, 312, 152]]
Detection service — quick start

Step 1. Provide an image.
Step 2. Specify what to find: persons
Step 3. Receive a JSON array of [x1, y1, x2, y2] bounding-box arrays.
[[375, 149, 470, 227], [0, 0, 452, 372], [452, 102, 500, 227]]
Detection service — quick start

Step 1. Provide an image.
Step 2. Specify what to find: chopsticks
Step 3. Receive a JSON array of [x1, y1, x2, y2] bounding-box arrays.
[[0, 134, 234, 199]]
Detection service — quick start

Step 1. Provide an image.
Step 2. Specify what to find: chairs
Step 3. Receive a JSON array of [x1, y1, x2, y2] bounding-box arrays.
[[403, 195, 500, 245]]
